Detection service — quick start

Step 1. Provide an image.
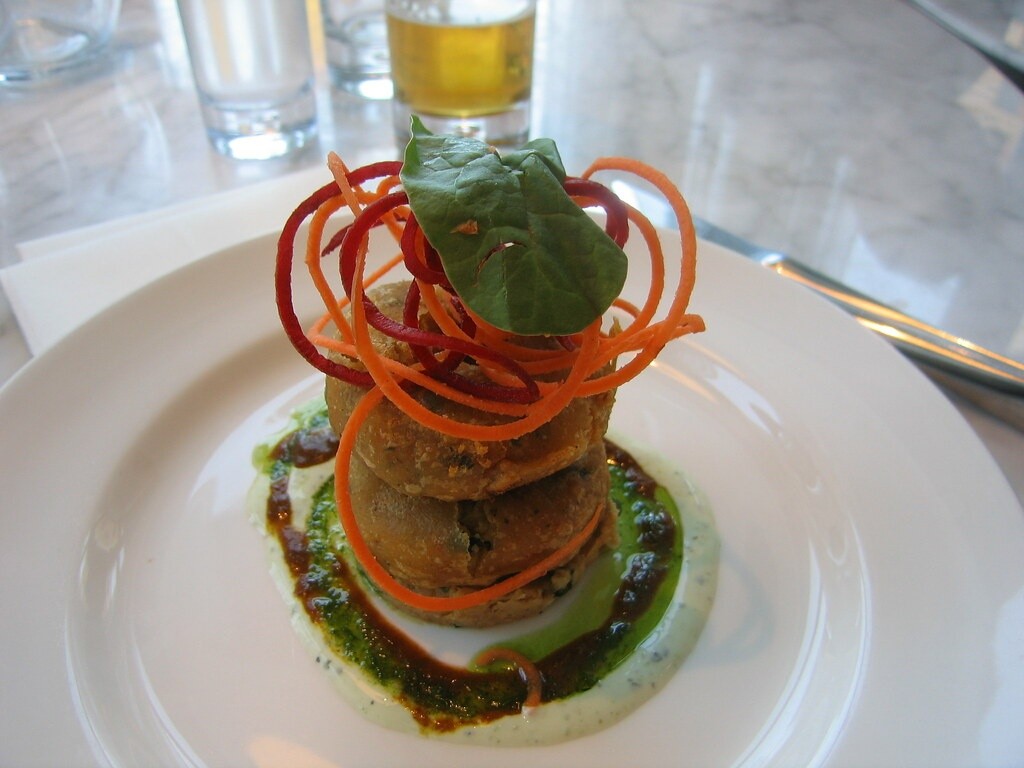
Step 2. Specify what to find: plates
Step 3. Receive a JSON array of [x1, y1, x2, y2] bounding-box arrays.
[[0, 204, 1020, 766]]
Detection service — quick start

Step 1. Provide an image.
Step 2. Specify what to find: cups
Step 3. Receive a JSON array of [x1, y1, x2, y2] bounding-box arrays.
[[152, 0, 317, 162], [383, 1, 535, 151], [1, 1, 121, 93], [320, 1, 451, 101]]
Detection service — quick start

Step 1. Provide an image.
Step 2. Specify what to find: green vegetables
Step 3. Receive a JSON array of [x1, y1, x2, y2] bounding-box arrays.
[[398, 115, 628, 336]]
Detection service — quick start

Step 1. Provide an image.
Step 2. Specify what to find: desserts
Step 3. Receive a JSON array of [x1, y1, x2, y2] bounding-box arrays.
[[324, 281, 616, 626]]
